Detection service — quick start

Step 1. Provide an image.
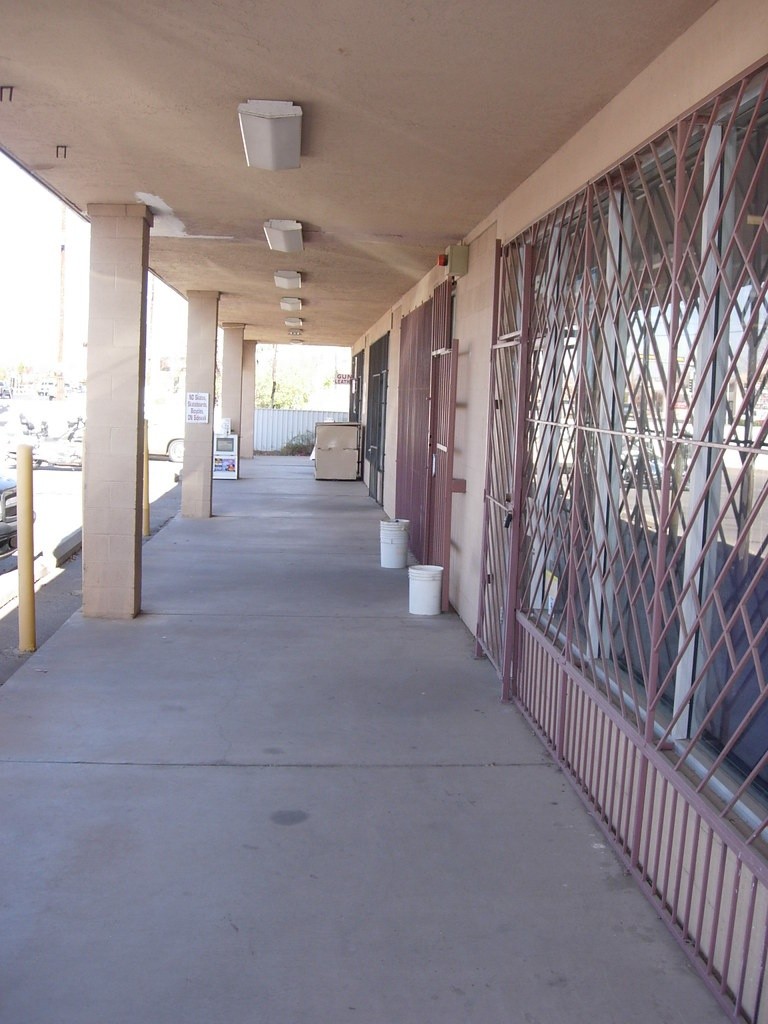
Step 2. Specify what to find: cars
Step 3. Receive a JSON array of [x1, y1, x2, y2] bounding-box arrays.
[[0, 475, 37, 561], [0, 381, 184, 472]]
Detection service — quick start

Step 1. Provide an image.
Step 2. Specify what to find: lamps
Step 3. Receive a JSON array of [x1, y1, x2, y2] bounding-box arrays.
[[280, 297, 301, 312], [285, 318, 304, 345], [237, 100, 303, 171], [274, 270, 301, 289], [263, 219, 304, 252]]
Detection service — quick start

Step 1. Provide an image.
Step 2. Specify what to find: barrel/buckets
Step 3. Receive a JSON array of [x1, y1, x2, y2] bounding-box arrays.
[[380, 519, 410, 568], [407, 565, 444, 616]]
